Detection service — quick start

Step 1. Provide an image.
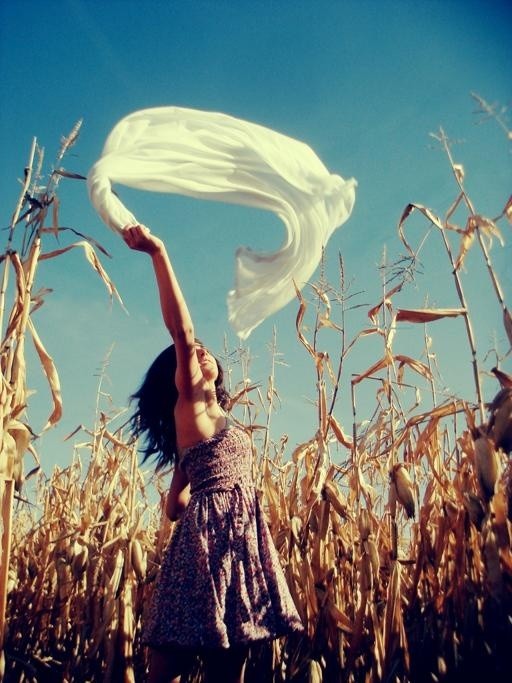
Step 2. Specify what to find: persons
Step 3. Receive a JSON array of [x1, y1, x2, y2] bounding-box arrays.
[[121, 223, 306, 682]]
